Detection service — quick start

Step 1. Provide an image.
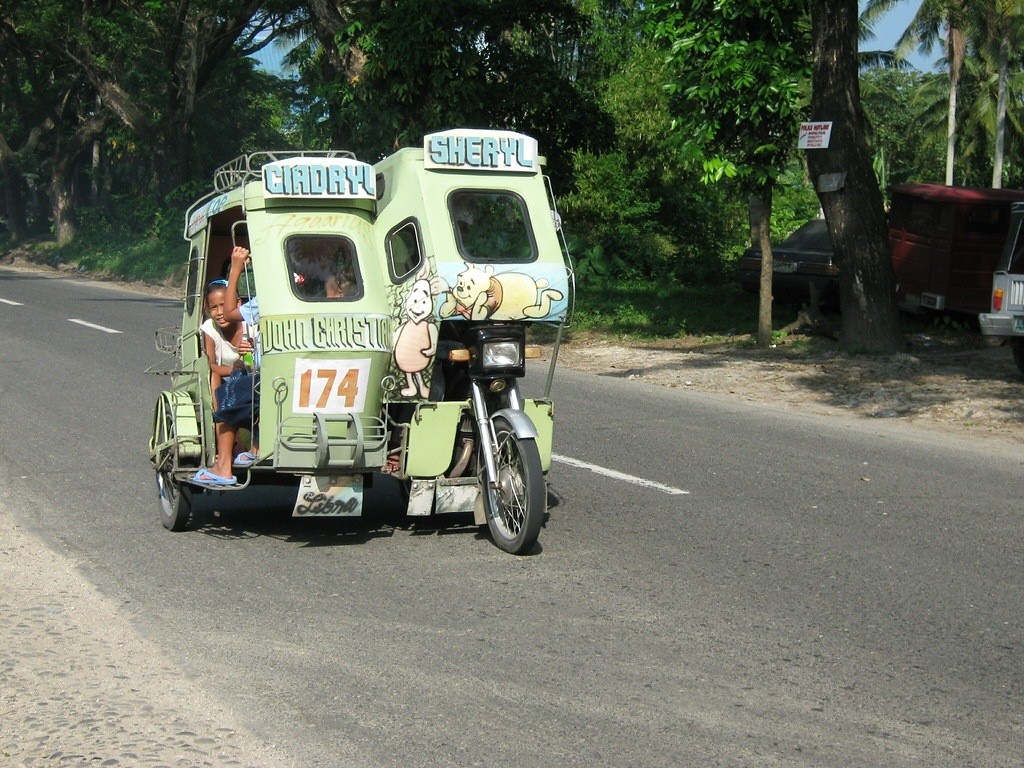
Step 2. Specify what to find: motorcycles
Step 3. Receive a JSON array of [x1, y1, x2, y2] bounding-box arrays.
[[149, 129, 575, 556]]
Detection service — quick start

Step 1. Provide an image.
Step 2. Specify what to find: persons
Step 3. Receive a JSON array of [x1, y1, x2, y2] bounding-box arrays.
[[191, 246, 328, 485], [200, 280, 252, 462]]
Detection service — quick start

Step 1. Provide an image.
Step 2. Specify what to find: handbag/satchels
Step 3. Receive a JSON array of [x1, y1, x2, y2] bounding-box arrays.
[[212, 369, 261, 434]]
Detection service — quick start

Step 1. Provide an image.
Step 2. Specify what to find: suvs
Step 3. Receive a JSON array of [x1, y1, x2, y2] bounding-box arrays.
[[976, 195, 1024, 377], [881, 186, 1023, 379]]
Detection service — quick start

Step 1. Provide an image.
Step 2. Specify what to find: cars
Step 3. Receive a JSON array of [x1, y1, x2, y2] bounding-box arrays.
[[734, 218, 838, 317]]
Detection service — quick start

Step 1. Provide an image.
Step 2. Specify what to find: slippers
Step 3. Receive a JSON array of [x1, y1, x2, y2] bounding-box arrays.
[[233, 451, 258, 465], [192, 468, 237, 484]]
